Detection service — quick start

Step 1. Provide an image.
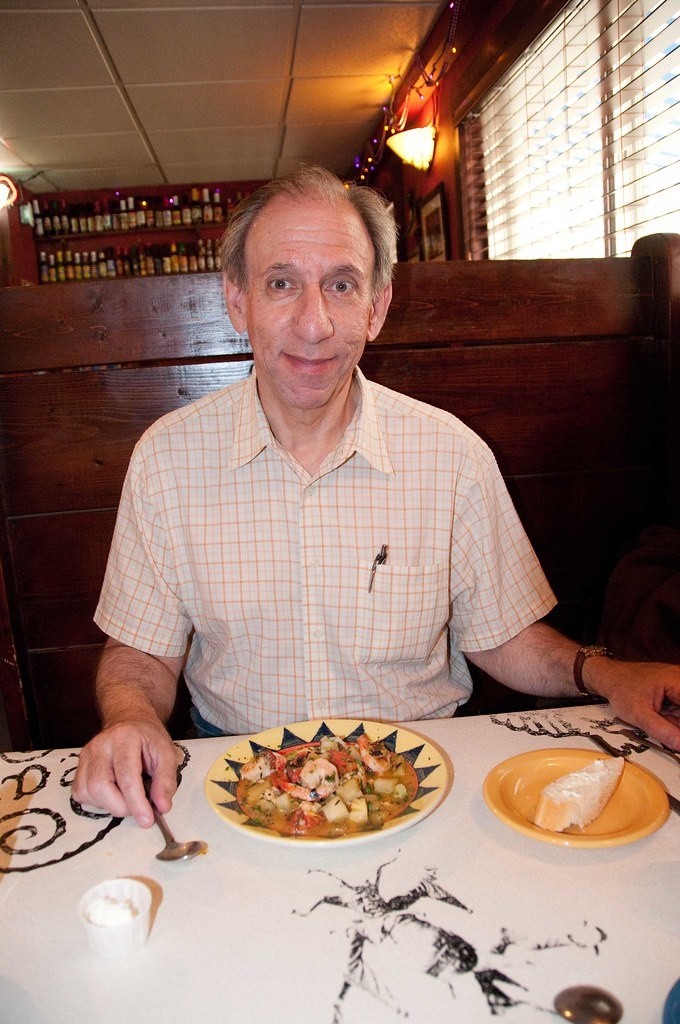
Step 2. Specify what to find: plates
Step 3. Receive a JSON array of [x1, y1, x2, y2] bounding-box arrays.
[[484, 748, 669, 847], [204, 719, 448, 846]]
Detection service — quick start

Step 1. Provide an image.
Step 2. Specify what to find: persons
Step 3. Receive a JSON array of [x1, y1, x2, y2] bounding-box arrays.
[[72, 165, 680, 828]]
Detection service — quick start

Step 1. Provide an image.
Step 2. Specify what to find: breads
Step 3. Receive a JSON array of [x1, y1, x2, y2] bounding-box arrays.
[[534, 757, 625, 833]]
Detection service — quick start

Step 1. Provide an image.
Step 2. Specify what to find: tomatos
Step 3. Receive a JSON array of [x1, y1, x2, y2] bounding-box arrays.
[[289, 808, 326, 830], [326, 749, 358, 774]]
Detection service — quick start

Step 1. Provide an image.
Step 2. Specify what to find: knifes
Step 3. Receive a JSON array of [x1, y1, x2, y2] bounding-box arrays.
[[588, 734, 680, 819]]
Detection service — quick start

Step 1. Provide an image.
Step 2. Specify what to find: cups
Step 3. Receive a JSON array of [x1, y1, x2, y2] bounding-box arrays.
[[77, 878, 152, 952]]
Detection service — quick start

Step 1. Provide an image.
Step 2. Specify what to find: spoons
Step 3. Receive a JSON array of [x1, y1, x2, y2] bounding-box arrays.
[[151, 800, 207, 863]]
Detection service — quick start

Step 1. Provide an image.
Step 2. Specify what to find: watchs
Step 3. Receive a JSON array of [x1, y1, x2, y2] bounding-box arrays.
[[574, 644, 615, 699]]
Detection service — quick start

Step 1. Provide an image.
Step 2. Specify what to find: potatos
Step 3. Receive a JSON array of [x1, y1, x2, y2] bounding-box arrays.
[[258, 738, 407, 824]]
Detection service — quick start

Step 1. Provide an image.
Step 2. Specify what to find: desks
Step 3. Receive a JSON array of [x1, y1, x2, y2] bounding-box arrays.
[[0, 690, 680, 1024]]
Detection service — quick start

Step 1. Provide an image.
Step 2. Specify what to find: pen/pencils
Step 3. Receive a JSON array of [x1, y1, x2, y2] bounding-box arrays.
[[368, 544, 389, 594]]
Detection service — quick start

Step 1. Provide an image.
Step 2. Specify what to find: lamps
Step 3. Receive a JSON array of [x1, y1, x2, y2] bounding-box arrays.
[[386, 121, 435, 174]]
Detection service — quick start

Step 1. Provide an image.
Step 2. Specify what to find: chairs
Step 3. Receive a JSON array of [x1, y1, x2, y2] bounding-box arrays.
[[0, 233, 680, 753]]
